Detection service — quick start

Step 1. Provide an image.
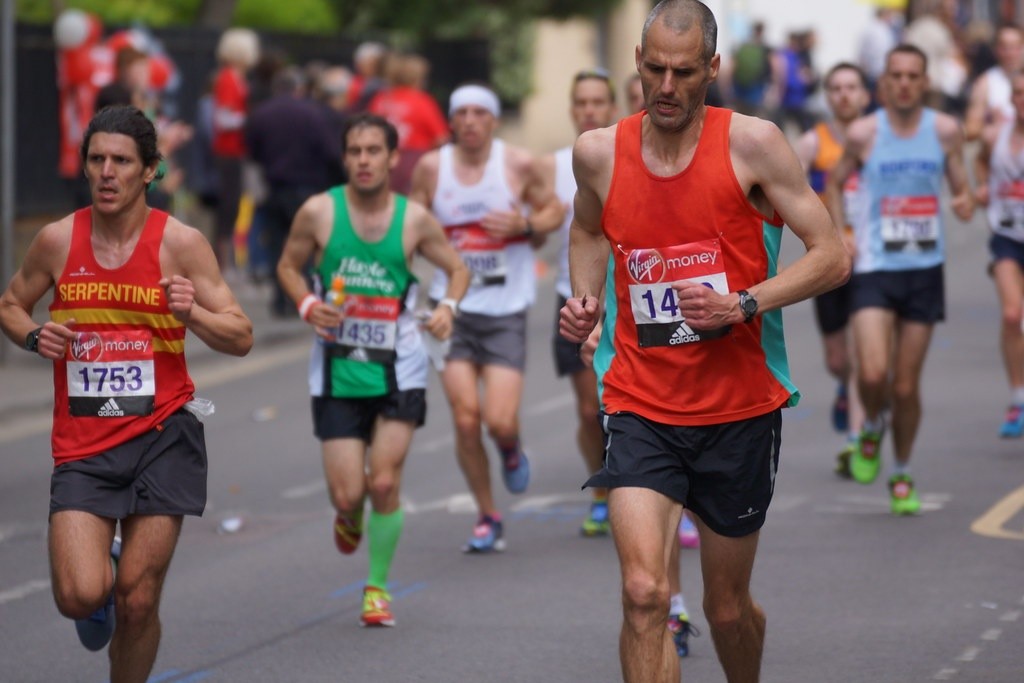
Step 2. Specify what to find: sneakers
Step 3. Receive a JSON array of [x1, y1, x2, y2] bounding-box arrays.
[[997, 403, 1024, 439], [887, 474, 918, 515], [332, 467, 369, 554], [462, 513, 507, 554], [666, 611, 699, 656], [75, 536, 121, 652], [496, 443, 530, 495], [849, 415, 885, 484], [678, 511, 700, 549], [831, 383, 850, 432], [579, 496, 610, 537], [357, 586, 396, 628]]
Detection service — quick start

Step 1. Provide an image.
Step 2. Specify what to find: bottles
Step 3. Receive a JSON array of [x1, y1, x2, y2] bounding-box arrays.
[[315, 276, 346, 346]]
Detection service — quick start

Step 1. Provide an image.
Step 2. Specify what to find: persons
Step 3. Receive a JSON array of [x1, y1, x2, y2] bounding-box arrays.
[[52, 8, 450, 323], [0, 103, 254, 683], [825, 43, 974, 519], [406, 83, 567, 556], [559, 0, 853, 683], [543, 68, 704, 550], [795, 0, 1024, 437], [275, 112, 473, 629]]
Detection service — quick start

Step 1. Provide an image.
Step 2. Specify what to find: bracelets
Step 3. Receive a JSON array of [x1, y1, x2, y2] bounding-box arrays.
[[297, 292, 323, 322], [521, 219, 534, 238], [441, 297, 461, 318]]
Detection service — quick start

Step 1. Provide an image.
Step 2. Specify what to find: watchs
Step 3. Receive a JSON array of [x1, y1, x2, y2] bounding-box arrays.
[[24, 326, 43, 353], [736, 289, 759, 324]]
